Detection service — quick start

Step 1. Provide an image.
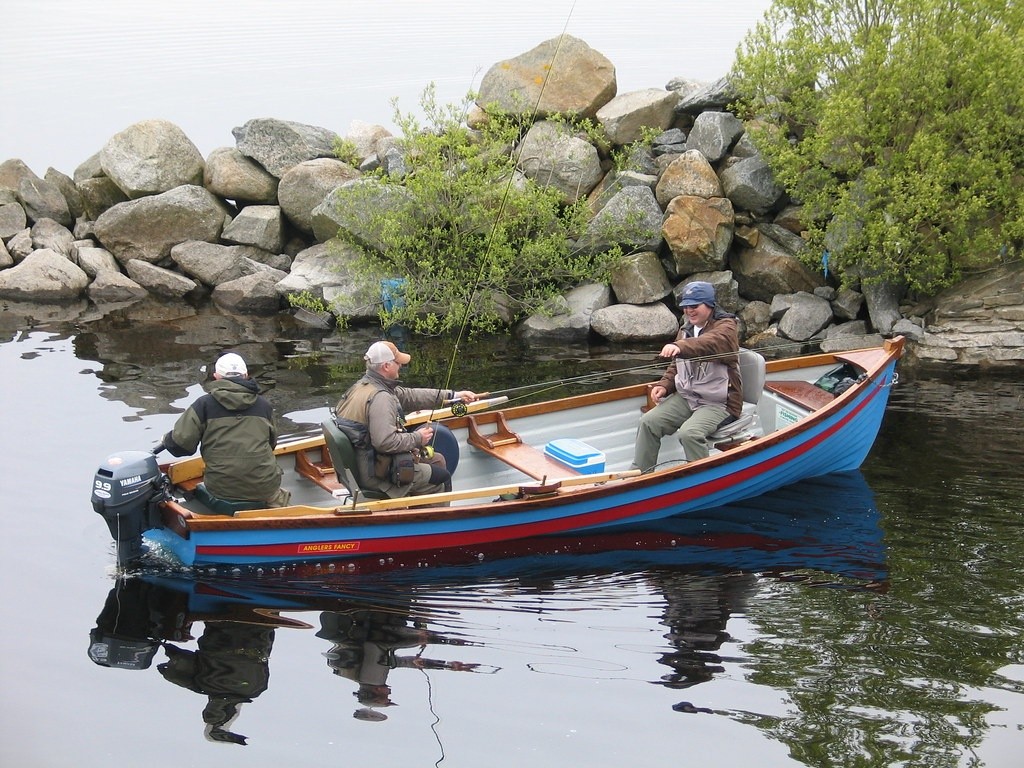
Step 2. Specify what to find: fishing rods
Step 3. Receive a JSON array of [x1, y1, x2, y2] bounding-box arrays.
[[420, 1, 578, 461], [441, 330, 914, 418]]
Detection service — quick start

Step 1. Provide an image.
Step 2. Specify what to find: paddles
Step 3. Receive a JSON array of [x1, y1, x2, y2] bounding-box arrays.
[[233, 468, 642, 519], [167, 394, 510, 485]]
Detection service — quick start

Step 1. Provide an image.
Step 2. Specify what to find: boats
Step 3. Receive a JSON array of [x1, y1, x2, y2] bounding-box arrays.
[[90, 334, 908, 567], [87, 466, 890, 675]]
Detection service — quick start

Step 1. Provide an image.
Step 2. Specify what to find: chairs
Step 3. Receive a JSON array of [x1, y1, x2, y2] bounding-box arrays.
[[653, 345, 766, 453]]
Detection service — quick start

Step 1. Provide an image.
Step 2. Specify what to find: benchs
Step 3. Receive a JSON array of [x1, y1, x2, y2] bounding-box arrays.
[[763, 380, 840, 412], [294, 452, 376, 502], [195, 483, 268, 516], [467, 410, 585, 484]]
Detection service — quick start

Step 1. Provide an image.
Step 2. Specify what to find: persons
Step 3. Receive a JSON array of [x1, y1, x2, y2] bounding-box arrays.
[[334, 339, 479, 509], [162, 352, 291, 507], [627, 280, 743, 475]]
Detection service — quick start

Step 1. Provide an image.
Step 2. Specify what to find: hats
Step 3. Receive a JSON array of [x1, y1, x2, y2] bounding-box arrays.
[[679, 281, 715, 308], [215, 352, 247, 379], [366, 340, 412, 365]]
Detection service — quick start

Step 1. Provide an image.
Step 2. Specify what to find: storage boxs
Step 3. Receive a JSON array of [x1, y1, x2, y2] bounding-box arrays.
[[543, 439, 607, 476]]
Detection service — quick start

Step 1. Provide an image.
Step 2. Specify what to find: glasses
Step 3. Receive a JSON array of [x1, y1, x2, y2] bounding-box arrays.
[[683, 302, 703, 309]]
